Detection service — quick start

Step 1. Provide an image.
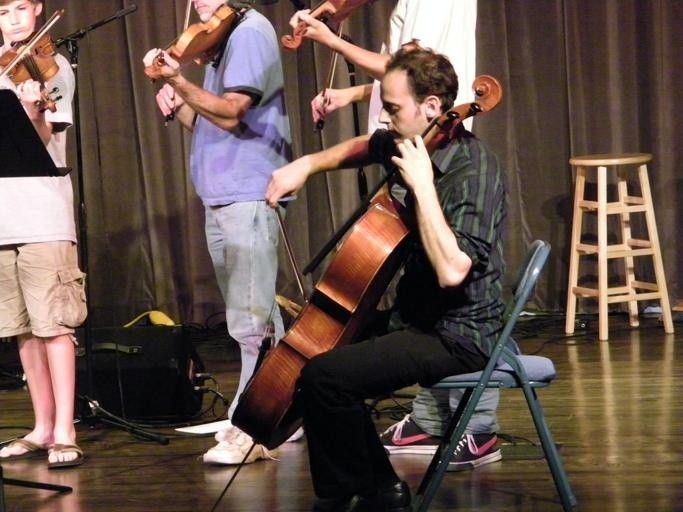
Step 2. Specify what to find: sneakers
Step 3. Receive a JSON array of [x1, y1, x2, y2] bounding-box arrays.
[[445, 431, 503, 472], [202, 425, 281, 466], [378, 413, 443, 455]]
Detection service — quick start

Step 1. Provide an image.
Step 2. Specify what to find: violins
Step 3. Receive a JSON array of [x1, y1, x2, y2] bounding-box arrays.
[[0, 31, 63, 113], [279, 1, 368, 52], [148, 5, 242, 84]]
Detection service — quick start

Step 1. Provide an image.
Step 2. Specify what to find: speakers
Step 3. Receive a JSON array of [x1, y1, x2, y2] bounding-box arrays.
[[70, 326, 205, 422]]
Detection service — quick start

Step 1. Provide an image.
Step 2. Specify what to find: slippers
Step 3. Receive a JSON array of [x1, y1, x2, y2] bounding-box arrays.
[[47, 443, 85, 468], [0, 438, 48, 461]]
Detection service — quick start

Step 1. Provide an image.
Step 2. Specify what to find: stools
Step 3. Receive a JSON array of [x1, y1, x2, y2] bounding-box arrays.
[[564, 152, 675, 341]]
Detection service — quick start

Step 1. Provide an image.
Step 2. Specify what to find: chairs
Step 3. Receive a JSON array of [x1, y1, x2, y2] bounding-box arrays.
[[411, 239, 578, 512]]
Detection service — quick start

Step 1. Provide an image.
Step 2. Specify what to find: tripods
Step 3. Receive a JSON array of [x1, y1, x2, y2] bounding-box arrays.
[[0, 5, 170, 447]]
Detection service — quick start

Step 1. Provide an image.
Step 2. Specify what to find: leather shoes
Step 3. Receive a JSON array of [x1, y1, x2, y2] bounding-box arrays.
[[312, 480, 413, 512]]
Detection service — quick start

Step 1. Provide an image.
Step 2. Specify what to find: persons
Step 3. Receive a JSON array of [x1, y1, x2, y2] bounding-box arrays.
[[1, 0, 88, 470], [201, 458, 278, 512], [140, 1, 302, 469], [286, 0, 505, 475], [263, 47, 507, 511], [33, 464, 81, 512]]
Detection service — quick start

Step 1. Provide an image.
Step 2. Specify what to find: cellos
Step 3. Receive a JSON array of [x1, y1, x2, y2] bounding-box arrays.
[[232, 75, 504, 450]]
